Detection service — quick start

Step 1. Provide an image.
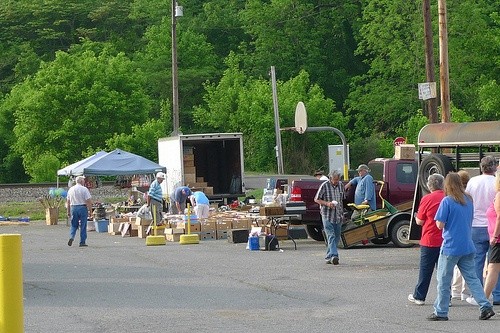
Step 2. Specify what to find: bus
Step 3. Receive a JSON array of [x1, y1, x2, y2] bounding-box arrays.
[[408, 121, 500, 242]]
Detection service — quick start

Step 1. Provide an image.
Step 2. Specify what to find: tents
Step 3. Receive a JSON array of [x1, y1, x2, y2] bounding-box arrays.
[[56, 148, 167, 226]]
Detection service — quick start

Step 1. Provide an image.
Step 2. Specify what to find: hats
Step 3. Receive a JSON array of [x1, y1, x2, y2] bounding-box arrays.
[[156, 172, 165, 178], [184, 188, 190, 196], [355, 165, 369, 171]]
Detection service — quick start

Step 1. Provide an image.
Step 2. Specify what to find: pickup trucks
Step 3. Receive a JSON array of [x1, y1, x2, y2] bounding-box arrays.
[[292, 158, 420, 249]]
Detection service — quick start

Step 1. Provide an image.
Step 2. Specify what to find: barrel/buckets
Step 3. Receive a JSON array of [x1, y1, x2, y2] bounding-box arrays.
[[265, 234, 276, 250], [248, 234, 260, 250]]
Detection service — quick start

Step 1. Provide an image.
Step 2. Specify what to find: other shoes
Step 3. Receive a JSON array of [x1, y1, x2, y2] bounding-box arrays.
[[479, 308, 495, 320], [332, 256, 339, 265], [80, 243, 88, 246], [326, 260, 339, 264], [68, 238, 73, 246], [408, 294, 425, 305], [426, 313, 448, 321], [493, 301, 500, 304], [449, 301, 452, 307], [466, 295, 493, 307]]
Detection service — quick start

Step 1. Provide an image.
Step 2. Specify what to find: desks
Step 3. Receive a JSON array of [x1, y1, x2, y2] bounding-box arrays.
[[252, 215, 297, 250]]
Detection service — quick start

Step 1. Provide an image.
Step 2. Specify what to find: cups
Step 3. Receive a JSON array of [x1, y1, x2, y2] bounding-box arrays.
[[331, 200, 339, 209]]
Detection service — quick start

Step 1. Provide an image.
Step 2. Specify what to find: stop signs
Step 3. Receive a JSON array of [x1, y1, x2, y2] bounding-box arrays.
[[393, 137, 405, 147]]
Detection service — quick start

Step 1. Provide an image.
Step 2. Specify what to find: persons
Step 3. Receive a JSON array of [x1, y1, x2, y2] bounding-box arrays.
[[451, 155, 500, 307], [170, 186, 191, 215], [67, 176, 92, 247], [426, 173, 495, 321], [190, 190, 210, 218], [408, 173, 452, 307], [147, 172, 165, 223], [344, 164, 376, 219], [314, 170, 329, 180], [314, 170, 346, 265]]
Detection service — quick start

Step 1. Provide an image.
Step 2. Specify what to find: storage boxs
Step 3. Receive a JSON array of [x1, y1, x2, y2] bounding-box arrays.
[[395, 144, 416, 160], [86, 147, 308, 240], [46, 208, 58, 225]]
